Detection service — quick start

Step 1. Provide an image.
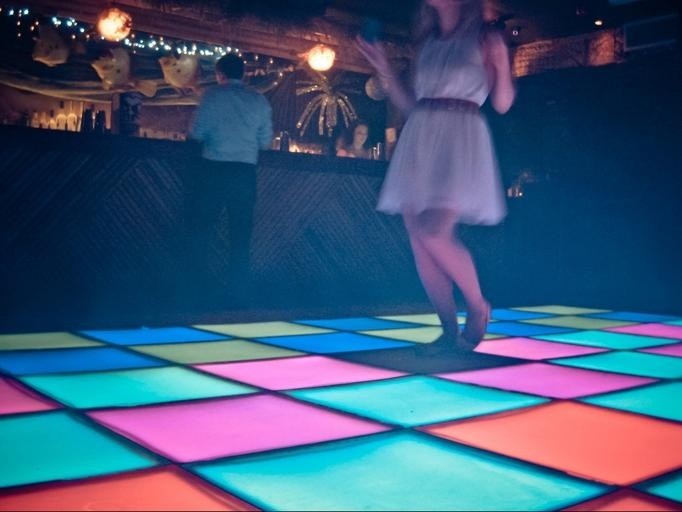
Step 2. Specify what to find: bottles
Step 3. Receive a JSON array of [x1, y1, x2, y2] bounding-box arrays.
[[280, 127, 289, 152], [4, 99, 107, 135]]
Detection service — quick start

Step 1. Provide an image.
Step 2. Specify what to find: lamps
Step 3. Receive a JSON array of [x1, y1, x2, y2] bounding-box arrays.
[[308, 41, 335, 71], [96, 1, 131, 44]]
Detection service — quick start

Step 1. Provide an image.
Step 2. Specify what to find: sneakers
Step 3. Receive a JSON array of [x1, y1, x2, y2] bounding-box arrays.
[[414, 333, 459, 358], [456, 304, 490, 358]]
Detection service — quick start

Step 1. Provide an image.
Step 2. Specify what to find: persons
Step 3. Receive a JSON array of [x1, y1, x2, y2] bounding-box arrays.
[[174, 51, 274, 307], [351, 0, 518, 357], [335, 120, 370, 160]]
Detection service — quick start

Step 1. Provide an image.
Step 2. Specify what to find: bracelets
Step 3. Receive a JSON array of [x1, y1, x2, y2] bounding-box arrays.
[[482, 16, 505, 32]]
